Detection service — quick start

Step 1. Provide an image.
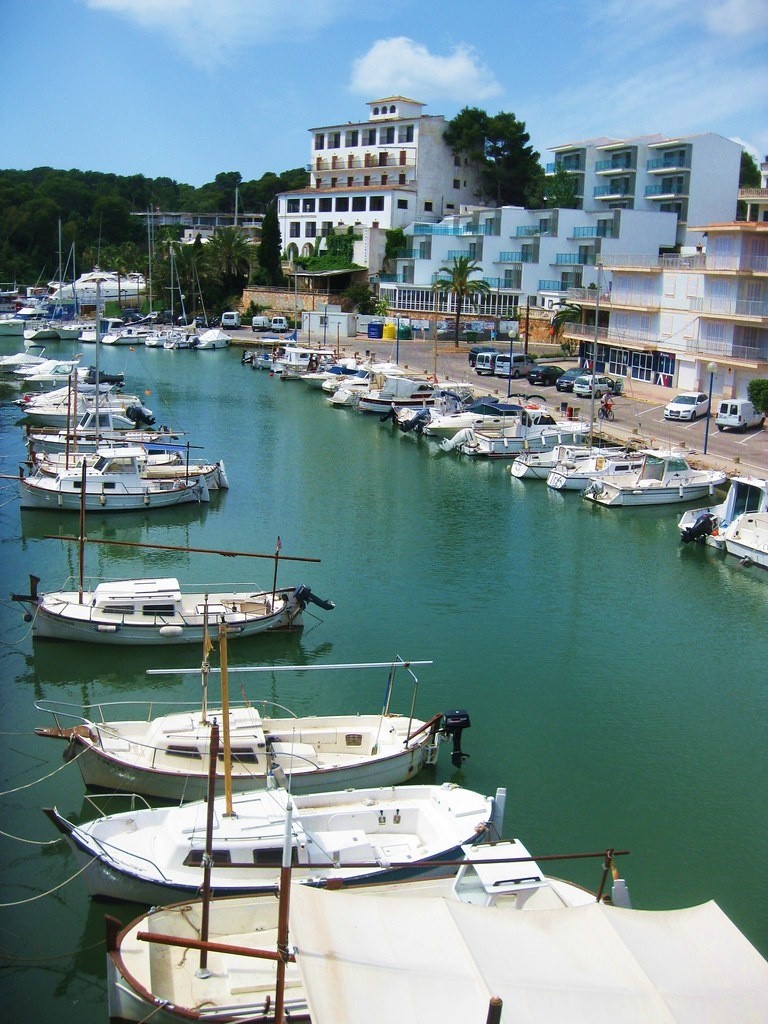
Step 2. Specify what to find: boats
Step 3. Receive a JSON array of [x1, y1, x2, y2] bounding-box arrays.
[[243, 341, 645, 490], [580, 448, 728, 506], [104, 718, 617, 1024], [40, 616, 506, 908], [678, 477, 768, 550], [725, 512, 768, 567], [0, 267, 235, 349], [9, 456, 322, 647], [33, 594, 473, 802], [0, 344, 231, 511]]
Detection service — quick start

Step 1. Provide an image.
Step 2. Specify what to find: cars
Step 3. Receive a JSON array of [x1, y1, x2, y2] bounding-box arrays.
[[663, 392, 709, 421], [526, 364, 565, 386], [555, 368, 592, 392], [121, 310, 290, 333]]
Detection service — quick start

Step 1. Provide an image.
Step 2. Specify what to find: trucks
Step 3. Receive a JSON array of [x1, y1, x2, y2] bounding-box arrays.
[[714, 399, 767, 434]]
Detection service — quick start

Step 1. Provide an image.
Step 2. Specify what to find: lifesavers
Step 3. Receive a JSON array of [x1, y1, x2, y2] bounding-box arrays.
[[117, 332, 121, 337], [428, 376, 438, 383], [167, 330, 172, 336], [525, 404, 538, 410]]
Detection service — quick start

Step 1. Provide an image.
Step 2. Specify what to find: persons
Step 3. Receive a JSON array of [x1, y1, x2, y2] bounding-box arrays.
[[601, 391, 614, 418]]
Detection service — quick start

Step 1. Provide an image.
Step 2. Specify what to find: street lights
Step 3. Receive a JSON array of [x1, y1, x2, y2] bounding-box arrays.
[[322, 302, 329, 345], [181, 294, 186, 326], [395, 313, 402, 365], [508, 330, 516, 397], [704, 361, 717, 454]]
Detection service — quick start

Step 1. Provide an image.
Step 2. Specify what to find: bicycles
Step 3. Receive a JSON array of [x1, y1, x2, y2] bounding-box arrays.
[[598, 403, 615, 422]]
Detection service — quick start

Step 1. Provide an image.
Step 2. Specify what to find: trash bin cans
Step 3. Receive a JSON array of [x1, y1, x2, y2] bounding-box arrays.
[[397, 326, 413, 340], [383, 323, 396, 338], [466, 332, 478, 341], [566, 407, 573, 420], [368, 324, 383, 338], [366, 350, 370, 356], [561, 403, 568, 411]]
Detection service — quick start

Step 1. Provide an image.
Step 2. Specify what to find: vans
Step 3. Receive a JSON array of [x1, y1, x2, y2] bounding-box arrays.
[[468, 346, 538, 379], [572, 374, 624, 398]]
